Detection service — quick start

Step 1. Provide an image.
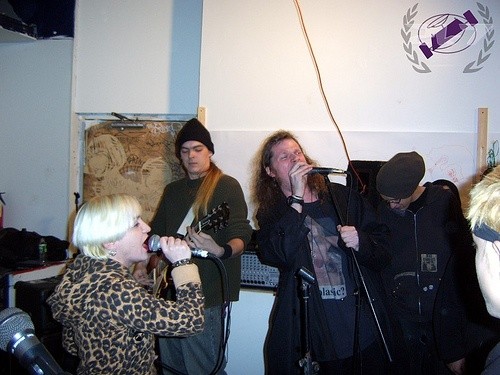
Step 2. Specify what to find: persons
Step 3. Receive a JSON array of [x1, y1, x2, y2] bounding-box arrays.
[[131, 118, 251, 375], [250, 129, 500, 375], [45, 191, 206, 375]]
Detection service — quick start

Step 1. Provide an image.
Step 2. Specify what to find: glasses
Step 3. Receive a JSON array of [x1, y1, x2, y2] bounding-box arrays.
[[385, 198, 402, 204]]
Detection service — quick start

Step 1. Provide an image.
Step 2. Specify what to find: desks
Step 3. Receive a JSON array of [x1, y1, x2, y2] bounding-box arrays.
[[7, 260, 71, 308]]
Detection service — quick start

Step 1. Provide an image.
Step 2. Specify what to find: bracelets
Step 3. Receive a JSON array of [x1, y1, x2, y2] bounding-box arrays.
[[220, 244, 233, 259], [292, 194, 303, 200], [171, 259, 193, 266]]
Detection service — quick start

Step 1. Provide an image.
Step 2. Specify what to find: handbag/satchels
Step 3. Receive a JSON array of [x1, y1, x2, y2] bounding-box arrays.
[[0, 227, 69, 270]]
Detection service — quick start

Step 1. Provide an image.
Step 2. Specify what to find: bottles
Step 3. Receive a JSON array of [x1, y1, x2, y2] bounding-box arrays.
[[38, 238, 47, 265]]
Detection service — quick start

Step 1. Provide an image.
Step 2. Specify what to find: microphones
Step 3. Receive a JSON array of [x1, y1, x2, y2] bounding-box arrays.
[[0, 307, 66, 375], [299, 166, 349, 174], [148, 234, 216, 258]]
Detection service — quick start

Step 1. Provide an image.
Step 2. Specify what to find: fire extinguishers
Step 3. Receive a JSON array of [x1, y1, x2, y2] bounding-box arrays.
[[0, 192, 6, 231]]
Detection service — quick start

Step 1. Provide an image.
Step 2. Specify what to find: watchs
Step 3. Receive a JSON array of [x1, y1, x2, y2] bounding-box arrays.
[[287, 195, 305, 207]]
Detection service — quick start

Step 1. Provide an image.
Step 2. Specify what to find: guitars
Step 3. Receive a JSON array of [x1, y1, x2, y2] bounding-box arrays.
[[145, 200, 231, 304]]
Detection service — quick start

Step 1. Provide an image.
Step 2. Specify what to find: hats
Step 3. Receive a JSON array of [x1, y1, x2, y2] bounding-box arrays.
[[375, 151, 425, 199], [175, 118, 214, 159]]
